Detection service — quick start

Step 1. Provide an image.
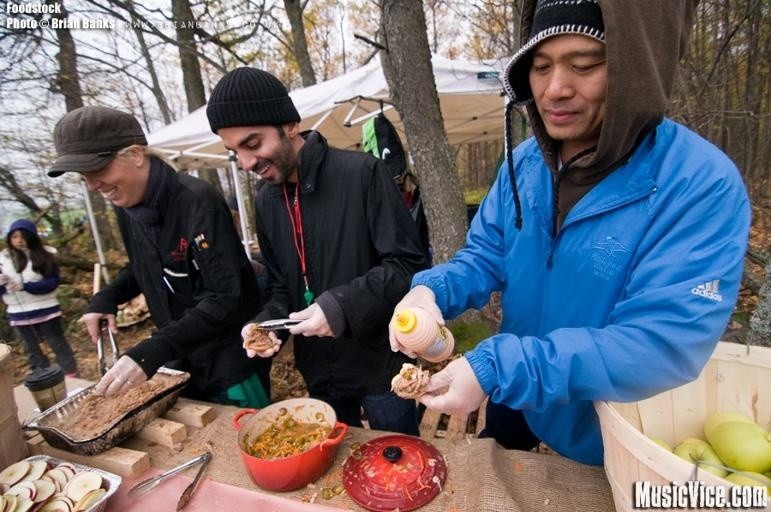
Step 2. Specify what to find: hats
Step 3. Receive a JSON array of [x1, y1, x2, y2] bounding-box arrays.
[[10, 219, 37, 235], [44, 104, 151, 176], [499, 0, 607, 106], [204, 66, 302, 131]]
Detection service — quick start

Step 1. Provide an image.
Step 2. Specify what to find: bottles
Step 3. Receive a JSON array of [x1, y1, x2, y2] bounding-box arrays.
[[393, 305, 456, 364]]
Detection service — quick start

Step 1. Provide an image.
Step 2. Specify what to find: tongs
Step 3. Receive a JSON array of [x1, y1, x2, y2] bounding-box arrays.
[[95, 327, 119, 378], [255, 318, 305, 331], [127, 452, 213, 510]]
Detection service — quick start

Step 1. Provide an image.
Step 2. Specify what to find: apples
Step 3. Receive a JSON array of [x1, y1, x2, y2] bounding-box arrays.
[[647, 412, 771, 497]]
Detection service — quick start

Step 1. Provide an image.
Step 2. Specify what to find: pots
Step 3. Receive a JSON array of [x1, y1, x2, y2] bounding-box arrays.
[[233, 397, 348, 492]]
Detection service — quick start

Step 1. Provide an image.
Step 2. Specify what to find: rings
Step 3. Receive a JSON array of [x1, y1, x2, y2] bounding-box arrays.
[[126, 380, 135, 385], [115, 378, 124, 383]]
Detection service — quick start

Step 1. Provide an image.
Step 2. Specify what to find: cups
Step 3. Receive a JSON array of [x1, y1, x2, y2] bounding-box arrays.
[[23, 366, 69, 413]]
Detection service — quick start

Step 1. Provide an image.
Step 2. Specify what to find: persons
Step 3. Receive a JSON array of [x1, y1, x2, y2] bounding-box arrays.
[[0, 218, 83, 378], [381, 0, 756, 470], [205, 66, 430, 437], [45, 105, 273, 410]]
